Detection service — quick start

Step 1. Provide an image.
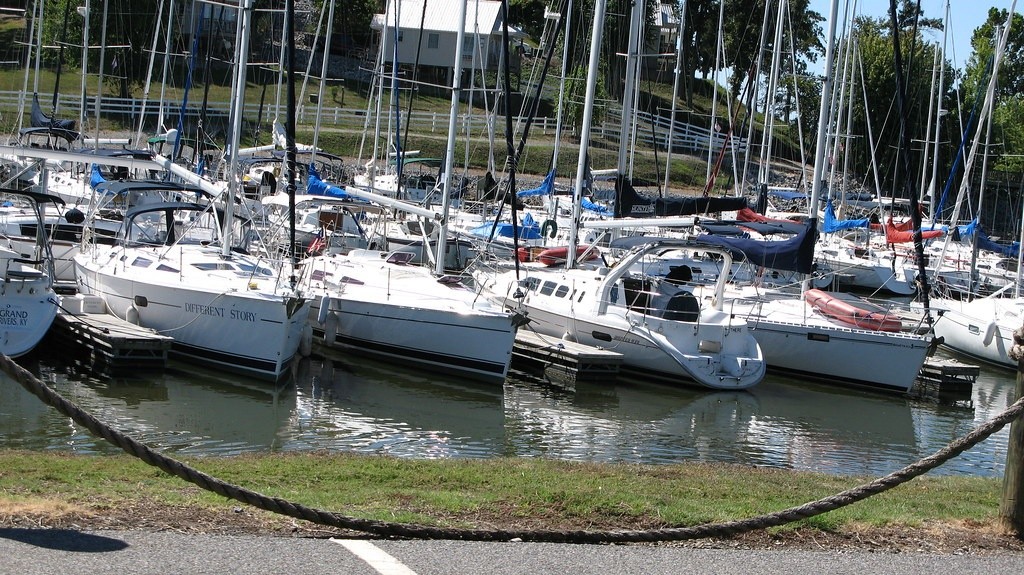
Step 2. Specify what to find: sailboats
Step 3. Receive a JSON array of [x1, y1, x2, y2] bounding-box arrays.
[[0, 0, 1024, 399]]
